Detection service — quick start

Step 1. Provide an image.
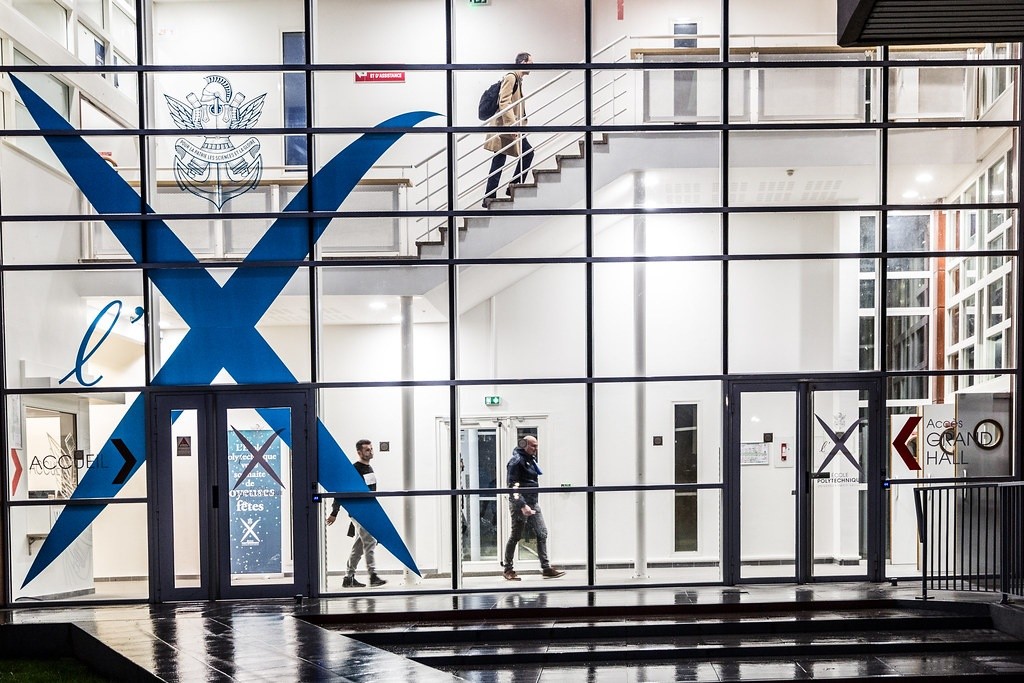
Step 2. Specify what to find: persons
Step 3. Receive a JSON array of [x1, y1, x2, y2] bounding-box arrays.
[[324, 439, 387, 588], [479, 52, 535, 208], [500, 435, 567, 582]]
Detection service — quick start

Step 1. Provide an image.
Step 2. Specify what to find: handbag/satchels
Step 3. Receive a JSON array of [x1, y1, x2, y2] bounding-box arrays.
[[348, 522, 381, 544]]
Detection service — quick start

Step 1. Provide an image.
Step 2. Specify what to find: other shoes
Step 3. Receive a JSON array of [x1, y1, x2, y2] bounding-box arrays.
[[370, 574, 386, 586], [502, 569, 521, 580], [506, 188, 511, 196], [343, 577, 365, 586], [483, 198, 488, 208], [542, 567, 566, 579]]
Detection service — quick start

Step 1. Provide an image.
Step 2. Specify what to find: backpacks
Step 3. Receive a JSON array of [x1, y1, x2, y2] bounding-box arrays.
[[478, 73, 518, 120]]
